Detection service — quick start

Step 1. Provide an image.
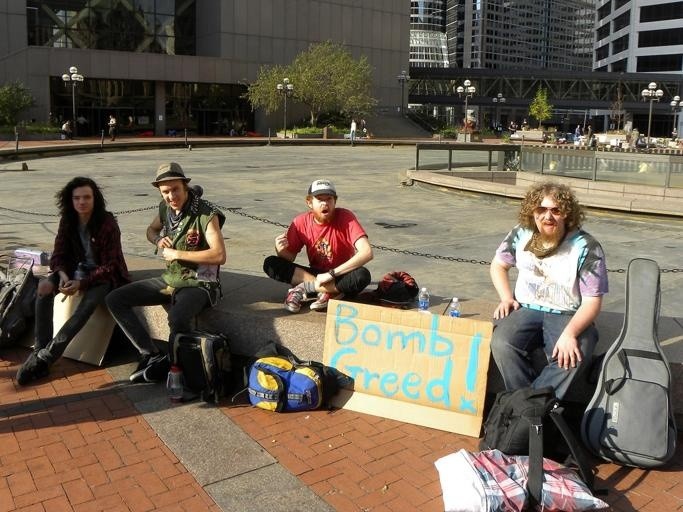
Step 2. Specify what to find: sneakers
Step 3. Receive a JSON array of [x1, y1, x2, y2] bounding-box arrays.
[[129, 352, 172, 384], [16, 346, 51, 386], [284, 286, 305, 313], [309, 291, 345, 310]]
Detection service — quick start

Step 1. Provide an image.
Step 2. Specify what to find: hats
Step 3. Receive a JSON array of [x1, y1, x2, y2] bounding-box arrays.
[[151, 163, 191, 188], [308, 179, 336, 197]]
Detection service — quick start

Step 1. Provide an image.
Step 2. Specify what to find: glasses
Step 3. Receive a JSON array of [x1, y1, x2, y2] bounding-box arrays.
[[534, 206, 561, 216]]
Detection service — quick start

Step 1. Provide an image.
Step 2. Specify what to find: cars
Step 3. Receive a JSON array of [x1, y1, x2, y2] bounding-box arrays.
[[488, 120, 575, 145], [636, 137, 656, 148]]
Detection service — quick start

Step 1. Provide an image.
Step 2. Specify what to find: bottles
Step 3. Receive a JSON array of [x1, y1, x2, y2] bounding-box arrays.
[[417, 286, 432, 313], [448, 296, 460, 318], [74, 262, 84, 280], [167, 367, 185, 403]]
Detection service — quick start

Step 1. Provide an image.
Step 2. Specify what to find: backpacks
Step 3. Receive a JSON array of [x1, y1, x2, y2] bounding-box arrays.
[[479, 386, 594, 508], [173, 329, 234, 405]]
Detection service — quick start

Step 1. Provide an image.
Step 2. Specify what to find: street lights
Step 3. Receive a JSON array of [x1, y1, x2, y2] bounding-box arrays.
[[276, 77, 293, 142], [62, 65, 85, 138], [491, 93, 508, 131], [669, 94, 683, 142], [457, 79, 475, 143], [640, 82, 662, 148], [395, 70, 411, 119]]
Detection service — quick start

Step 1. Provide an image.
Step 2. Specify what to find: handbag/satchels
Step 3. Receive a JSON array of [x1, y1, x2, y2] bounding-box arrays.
[[248, 355, 327, 412]]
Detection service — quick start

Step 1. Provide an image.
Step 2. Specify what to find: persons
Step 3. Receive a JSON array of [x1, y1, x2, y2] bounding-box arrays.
[[230, 126, 237, 136], [13, 172, 129, 388], [358, 116, 368, 139], [60, 119, 73, 139], [107, 114, 116, 142], [239, 126, 247, 136], [496, 118, 678, 149], [262, 179, 375, 312], [350, 120, 356, 141], [488, 182, 610, 403], [101, 162, 227, 389], [167, 127, 176, 137]]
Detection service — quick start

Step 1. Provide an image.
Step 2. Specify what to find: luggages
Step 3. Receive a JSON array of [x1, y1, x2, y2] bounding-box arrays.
[[0, 254, 37, 349]]
